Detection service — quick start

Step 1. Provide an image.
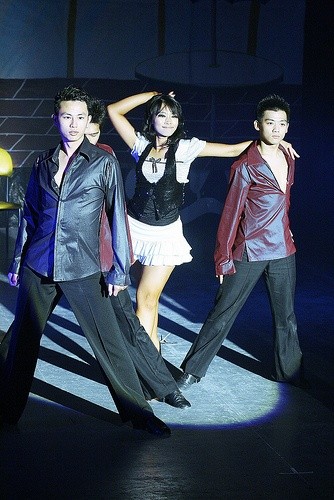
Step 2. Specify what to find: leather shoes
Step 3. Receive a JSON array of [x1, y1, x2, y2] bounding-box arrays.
[[164, 392, 191, 409], [288, 378, 311, 392], [141, 415, 171, 439], [176, 372, 200, 391], [0, 423, 17, 435]]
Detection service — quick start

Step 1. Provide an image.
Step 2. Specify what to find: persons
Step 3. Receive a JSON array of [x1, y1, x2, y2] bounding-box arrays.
[[0, 97, 191, 409], [107, 90, 300, 354], [175, 94, 303, 391], [0, 85, 170, 439]]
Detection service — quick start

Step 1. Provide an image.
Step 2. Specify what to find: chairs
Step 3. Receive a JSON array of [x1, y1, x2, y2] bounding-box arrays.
[[0, 149, 23, 275]]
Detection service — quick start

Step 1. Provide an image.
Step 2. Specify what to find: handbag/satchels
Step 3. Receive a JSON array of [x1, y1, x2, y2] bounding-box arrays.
[[7, 175, 28, 226]]
